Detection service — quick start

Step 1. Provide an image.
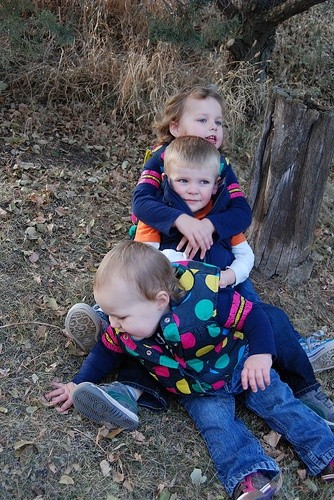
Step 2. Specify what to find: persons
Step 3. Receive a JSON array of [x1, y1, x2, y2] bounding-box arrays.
[[66, 86, 334, 371], [47, 240, 334, 500], [71, 135, 334, 430]]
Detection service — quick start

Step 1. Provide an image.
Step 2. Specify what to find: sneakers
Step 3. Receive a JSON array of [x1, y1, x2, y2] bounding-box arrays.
[[320, 458, 334, 480], [64, 303, 110, 354], [298, 385, 334, 430], [232, 471, 278, 500], [71, 381, 140, 431], [298, 337, 334, 373]]
